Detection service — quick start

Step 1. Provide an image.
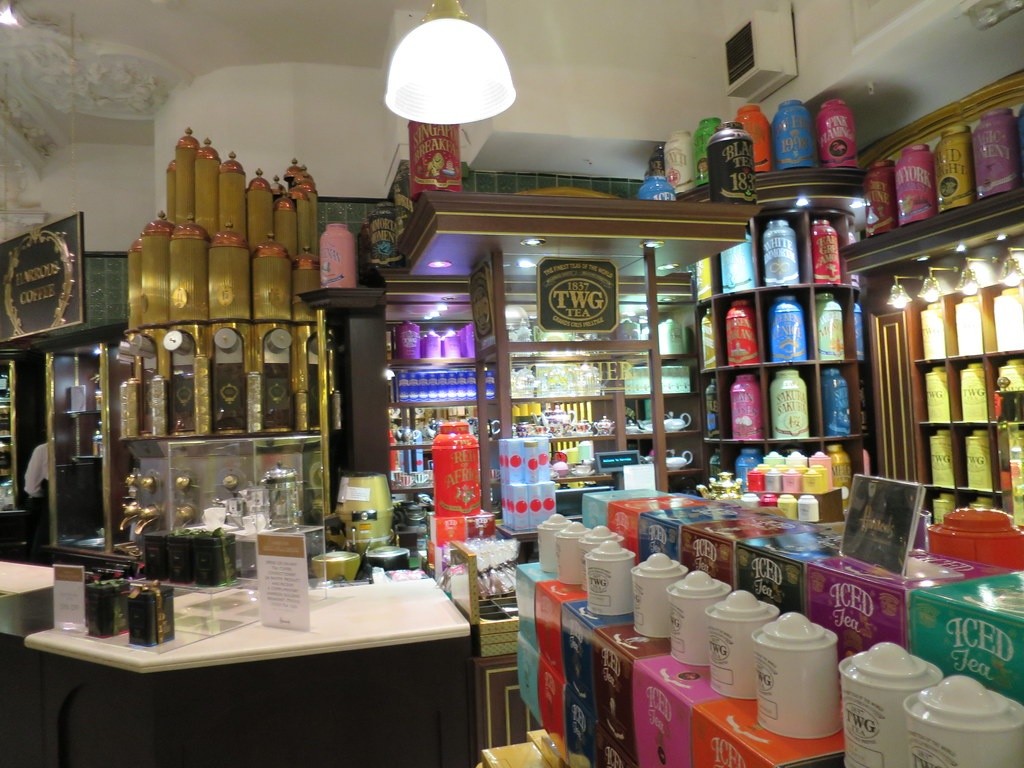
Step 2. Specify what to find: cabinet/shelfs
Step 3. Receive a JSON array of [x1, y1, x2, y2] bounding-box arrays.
[[903, 273, 1024, 509], [166, 434, 327, 604], [382, 269, 707, 524], [45, 343, 139, 566], [0, 358, 19, 510], [674, 165, 875, 478], [0, 509, 31, 563]]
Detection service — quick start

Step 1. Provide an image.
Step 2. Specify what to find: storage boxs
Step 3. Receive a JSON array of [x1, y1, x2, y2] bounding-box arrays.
[[472, 631, 518, 646], [561, 599, 634, 722], [515, 633, 544, 727], [192, 531, 237, 587], [565, 685, 595, 768], [83, 577, 129, 639], [166, 529, 201, 584], [607, 496, 715, 566], [592, 624, 671, 768], [638, 505, 745, 565], [143, 530, 176, 581], [582, 489, 684, 530], [449, 540, 519, 635], [692, 697, 846, 768], [624, 365, 692, 396], [538, 656, 568, 768], [471, 642, 518, 658], [635, 655, 724, 768], [734, 529, 843, 618], [534, 579, 587, 685], [595, 721, 638, 768], [681, 514, 831, 592], [744, 487, 845, 523], [907, 570, 1024, 706], [516, 562, 558, 655], [126, 584, 174, 649], [806, 549, 1020, 679]]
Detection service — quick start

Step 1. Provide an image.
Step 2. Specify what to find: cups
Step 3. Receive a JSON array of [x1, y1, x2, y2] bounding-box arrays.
[[393, 470, 434, 487], [509, 317, 602, 397]]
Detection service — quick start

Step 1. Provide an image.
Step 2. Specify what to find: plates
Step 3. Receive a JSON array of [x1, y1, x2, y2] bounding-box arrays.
[[572, 470, 595, 476]]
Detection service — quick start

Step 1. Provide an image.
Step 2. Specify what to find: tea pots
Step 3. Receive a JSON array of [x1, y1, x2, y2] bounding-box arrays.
[[392, 404, 616, 445]]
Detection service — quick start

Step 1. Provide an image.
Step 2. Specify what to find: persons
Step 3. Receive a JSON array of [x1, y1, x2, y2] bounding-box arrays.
[[22, 439, 49, 499]]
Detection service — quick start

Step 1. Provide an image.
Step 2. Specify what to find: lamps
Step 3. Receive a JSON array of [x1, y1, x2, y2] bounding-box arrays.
[[995, 247, 1024, 288], [885, 275, 922, 309], [917, 267, 956, 304], [955, 257, 989, 296], [384, 0, 517, 126]]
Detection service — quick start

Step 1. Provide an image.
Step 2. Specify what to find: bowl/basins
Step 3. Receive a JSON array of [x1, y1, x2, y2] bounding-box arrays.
[[554, 469, 570, 476], [575, 465, 591, 473]]
